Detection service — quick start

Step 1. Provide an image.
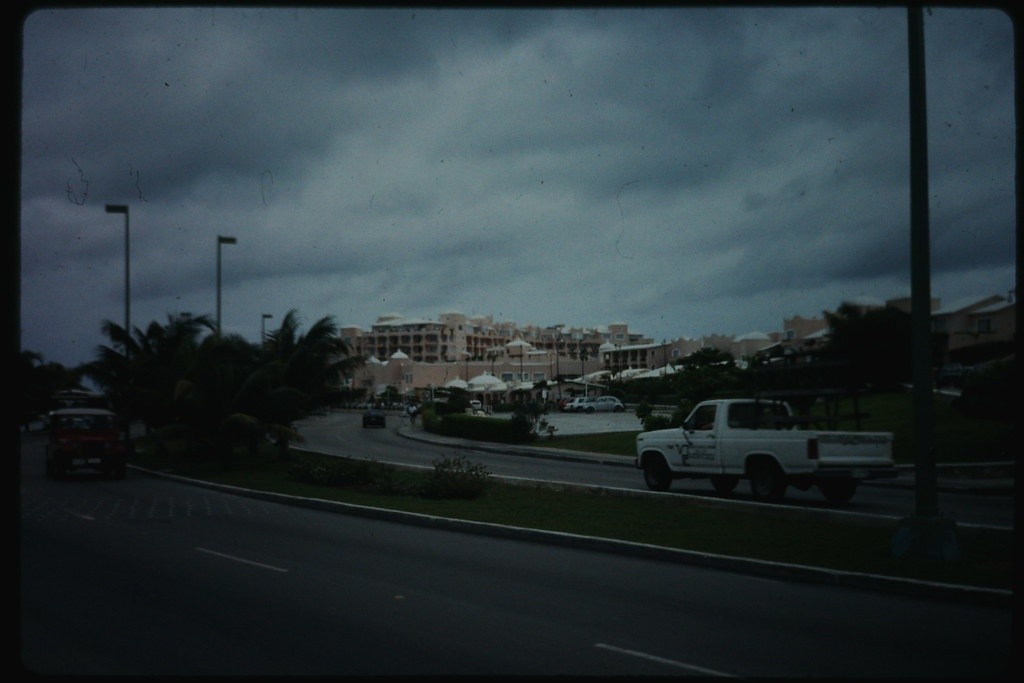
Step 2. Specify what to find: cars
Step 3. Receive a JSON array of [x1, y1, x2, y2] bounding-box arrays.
[[469, 399, 482, 410], [363, 411, 386, 429], [347, 399, 413, 410], [558, 397, 596, 413], [584, 396, 624, 413]]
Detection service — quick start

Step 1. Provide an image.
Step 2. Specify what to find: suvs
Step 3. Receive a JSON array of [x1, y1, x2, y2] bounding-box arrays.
[[47, 408, 127, 481]]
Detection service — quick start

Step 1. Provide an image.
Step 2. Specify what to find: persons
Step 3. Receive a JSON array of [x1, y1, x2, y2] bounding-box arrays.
[[339, 399, 418, 427]]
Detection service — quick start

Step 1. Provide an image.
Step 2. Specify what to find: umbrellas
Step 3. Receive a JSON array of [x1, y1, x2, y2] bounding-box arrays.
[[731, 332, 772, 343], [801, 326, 830, 339], [505, 333, 533, 382], [525, 346, 548, 356], [447, 363, 687, 406]]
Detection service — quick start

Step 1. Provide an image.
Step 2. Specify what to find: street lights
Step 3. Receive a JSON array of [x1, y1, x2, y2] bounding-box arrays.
[[261, 314, 272, 347], [105, 203, 131, 349], [215, 235, 237, 336]]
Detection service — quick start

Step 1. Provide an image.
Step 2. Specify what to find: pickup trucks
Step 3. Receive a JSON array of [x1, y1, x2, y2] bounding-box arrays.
[[635, 397, 899, 508]]
